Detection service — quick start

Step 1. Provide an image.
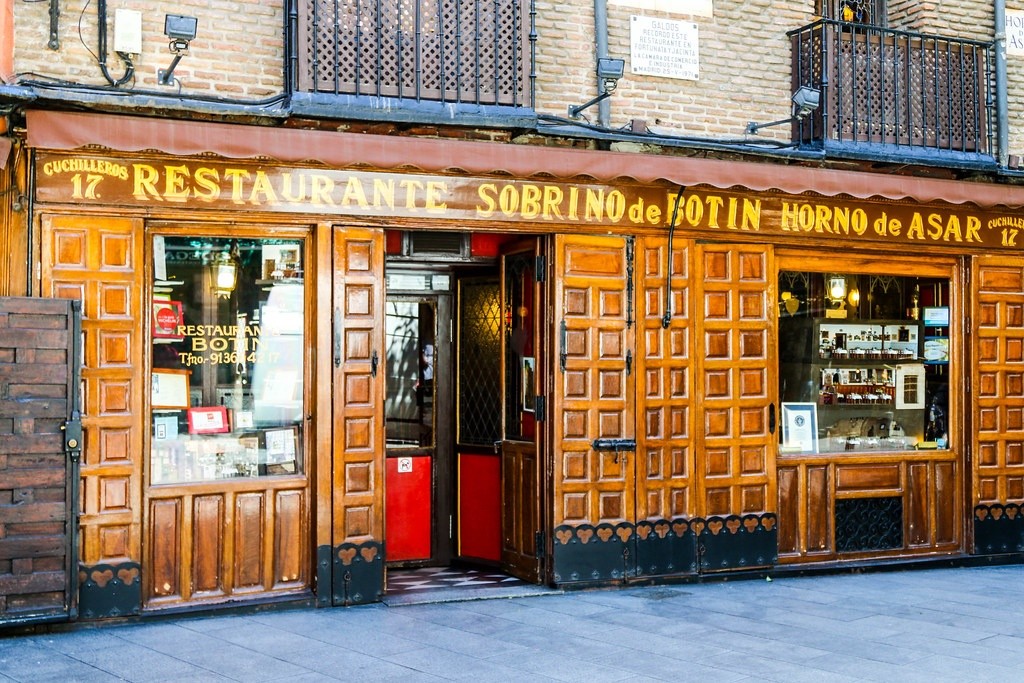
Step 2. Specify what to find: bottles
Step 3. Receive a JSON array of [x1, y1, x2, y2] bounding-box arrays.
[[849, 331, 890, 341], [926, 397, 943, 442], [909, 277, 920, 320]]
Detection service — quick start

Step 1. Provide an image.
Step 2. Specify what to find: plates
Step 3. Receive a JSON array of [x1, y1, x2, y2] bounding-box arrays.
[[925, 350, 946, 360]]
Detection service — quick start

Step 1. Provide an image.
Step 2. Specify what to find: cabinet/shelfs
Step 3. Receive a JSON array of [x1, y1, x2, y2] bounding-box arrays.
[[780, 317, 925, 443]]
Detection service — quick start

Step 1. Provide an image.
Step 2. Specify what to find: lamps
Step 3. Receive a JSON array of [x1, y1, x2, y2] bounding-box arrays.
[[159, 13, 198, 82], [746, 85, 821, 134], [823, 274, 848, 318], [568, 56, 627, 117], [210, 251, 240, 298]]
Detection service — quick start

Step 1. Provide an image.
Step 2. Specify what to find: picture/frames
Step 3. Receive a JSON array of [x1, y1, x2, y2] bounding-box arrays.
[[187, 406, 229, 435]]
